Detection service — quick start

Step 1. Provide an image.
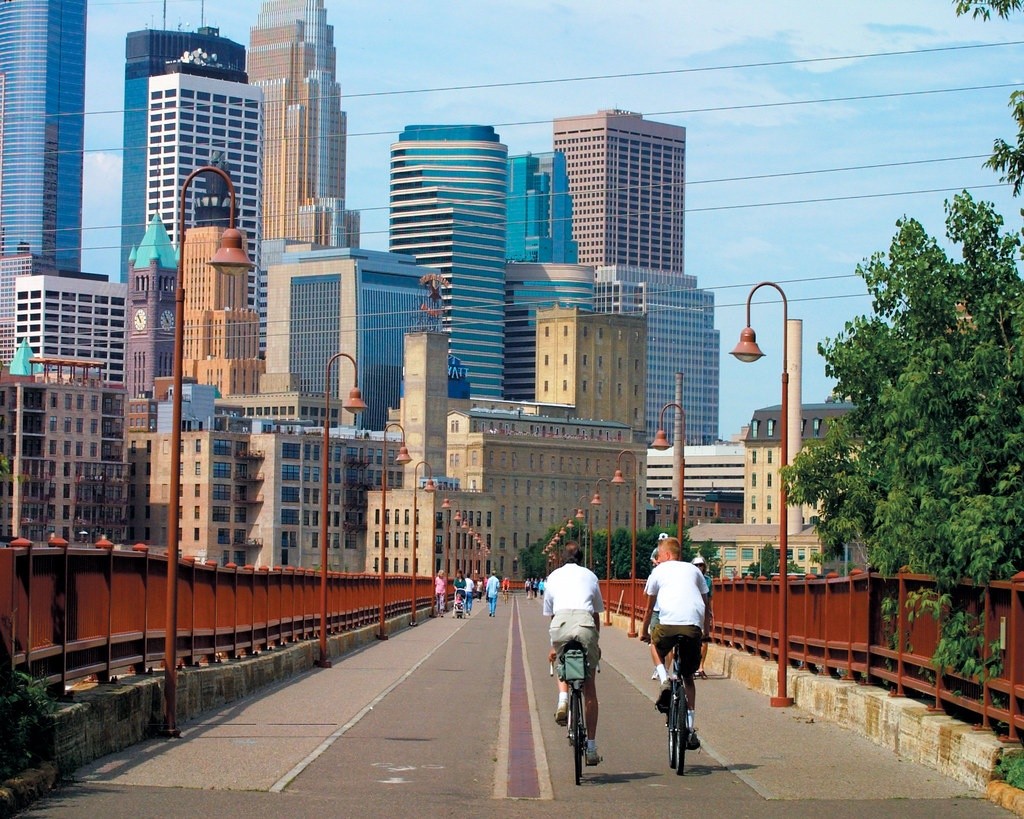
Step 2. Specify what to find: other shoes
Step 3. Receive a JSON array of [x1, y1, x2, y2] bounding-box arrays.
[[687, 734, 699, 750], [556, 704, 567, 727], [587, 754, 599, 765], [657, 680, 671, 713]]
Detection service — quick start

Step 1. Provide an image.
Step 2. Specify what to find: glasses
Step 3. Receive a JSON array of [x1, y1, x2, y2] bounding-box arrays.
[[695, 564, 703, 567]]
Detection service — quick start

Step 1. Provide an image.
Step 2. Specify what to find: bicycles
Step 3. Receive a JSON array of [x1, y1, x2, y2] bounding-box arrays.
[[549, 641, 598, 786], [641, 637, 693, 776], [502, 590, 508, 606]]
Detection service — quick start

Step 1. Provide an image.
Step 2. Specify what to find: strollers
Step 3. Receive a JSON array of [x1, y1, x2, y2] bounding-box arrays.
[[453, 587, 467, 619]]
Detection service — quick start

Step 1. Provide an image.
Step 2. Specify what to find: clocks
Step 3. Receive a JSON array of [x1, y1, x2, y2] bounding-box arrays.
[[161, 310, 175, 332], [135, 309, 146, 331]]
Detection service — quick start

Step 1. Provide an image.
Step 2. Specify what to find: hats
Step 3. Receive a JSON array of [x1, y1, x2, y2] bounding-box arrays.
[[658, 533, 668, 540], [692, 558, 705, 565]]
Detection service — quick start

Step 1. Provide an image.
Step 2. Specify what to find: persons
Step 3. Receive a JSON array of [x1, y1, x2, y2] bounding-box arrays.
[[543, 540, 605, 766], [643, 574, 674, 681], [688, 557, 715, 679], [434, 570, 546, 618], [650, 533, 668, 569], [640, 538, 710, 750]]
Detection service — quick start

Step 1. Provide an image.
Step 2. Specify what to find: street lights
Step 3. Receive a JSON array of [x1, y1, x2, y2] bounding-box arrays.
[[412, 459, 438, 623], [542, 496, 590, 571], [652, 403, 684, 562], [431, 497, 493, 620], [730, 283, 790, 706], [320, 353, 369, 669], [377, 423, 413, 638], [612, 449, 637, 639], [591, 476, 611, 625], [165, 167, 253, 738]]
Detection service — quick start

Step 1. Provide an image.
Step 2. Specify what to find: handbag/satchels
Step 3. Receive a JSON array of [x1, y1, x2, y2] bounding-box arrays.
[[473, 593, 477, 597]]
